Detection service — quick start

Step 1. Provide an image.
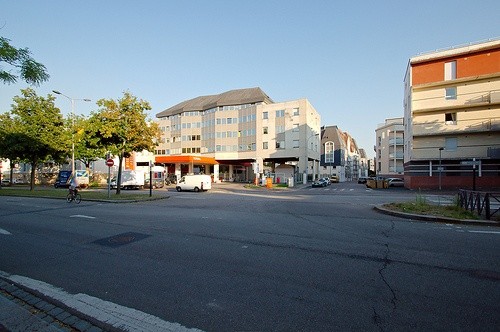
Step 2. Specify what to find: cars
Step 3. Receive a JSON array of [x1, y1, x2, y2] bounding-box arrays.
[[358, 178, 367, 184], [385, 178, 404, 188], [311, 177, 331, 188], [1, 177, 30, 184]]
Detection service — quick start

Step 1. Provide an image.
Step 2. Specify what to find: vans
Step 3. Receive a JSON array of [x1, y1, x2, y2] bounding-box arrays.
[[55, 170, 90, 188], [330, 176, 339, 183], [175, 174, 211, 191]]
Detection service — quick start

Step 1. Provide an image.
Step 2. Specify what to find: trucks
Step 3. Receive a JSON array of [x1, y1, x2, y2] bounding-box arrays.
[[108, 169, 145, 191]]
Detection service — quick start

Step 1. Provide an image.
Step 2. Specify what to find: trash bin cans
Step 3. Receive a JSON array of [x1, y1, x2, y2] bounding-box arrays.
[[376, 176, 383, 188], [366, 180, 376, 188], [384, 180, 388, 188]]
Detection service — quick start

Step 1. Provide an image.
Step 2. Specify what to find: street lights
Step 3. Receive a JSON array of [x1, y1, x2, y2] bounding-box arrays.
[[53, 89, 93, 175]]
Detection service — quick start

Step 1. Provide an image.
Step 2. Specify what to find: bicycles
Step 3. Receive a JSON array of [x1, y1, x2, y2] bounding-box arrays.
[[66, 187, 82, 204]]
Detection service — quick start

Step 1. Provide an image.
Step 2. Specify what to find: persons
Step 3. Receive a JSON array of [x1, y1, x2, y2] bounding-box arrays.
[[66, 171, 79, 201]]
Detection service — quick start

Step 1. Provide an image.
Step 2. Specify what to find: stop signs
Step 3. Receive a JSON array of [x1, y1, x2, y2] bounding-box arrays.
[[106, 159, 114, 166]]
